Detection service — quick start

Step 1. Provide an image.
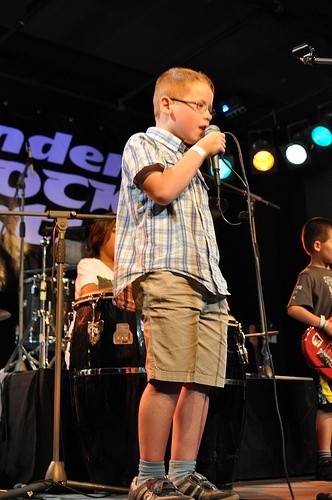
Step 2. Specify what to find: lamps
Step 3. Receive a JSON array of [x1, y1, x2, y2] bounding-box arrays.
[[311, 124, 332, 147], [208, 147, 234, 179], [251, 137, 275, 171], [286, 132, 308, 165]]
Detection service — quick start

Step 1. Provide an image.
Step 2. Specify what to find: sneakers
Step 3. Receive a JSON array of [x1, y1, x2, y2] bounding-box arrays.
[[128, 475, 195, 500], [172, 471, 239, 500]]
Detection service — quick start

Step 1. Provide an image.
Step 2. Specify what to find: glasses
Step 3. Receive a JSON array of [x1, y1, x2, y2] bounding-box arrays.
[[171, 98, 217, 117]]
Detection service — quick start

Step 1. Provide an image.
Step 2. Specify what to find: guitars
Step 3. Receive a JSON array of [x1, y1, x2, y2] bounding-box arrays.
[[302, 316, 332, 382]]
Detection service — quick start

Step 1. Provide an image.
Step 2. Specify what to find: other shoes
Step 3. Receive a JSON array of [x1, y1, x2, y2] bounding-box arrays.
[[313, 459, 332, 481]]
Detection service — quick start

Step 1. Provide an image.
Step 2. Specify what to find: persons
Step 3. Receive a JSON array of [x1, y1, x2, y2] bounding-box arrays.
[[65, 213, 116, 371], [287, 217, 332, 482], [113, 67, 240, 500]]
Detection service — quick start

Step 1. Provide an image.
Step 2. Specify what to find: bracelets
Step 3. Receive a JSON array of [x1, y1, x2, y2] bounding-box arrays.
[[190, 145, 207, 160]]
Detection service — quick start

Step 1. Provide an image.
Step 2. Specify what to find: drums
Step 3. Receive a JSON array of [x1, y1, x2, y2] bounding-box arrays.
[[22, 276, 72, 349], [70, 292, 262, 386]]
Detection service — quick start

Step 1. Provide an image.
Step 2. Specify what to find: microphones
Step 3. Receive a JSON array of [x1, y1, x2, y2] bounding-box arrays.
[[203, 125, 220, 186], [27, 141, 34, 175]]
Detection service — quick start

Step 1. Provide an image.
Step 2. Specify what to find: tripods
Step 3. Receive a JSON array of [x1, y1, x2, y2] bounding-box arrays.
[[0, 211, 128, 500]]
